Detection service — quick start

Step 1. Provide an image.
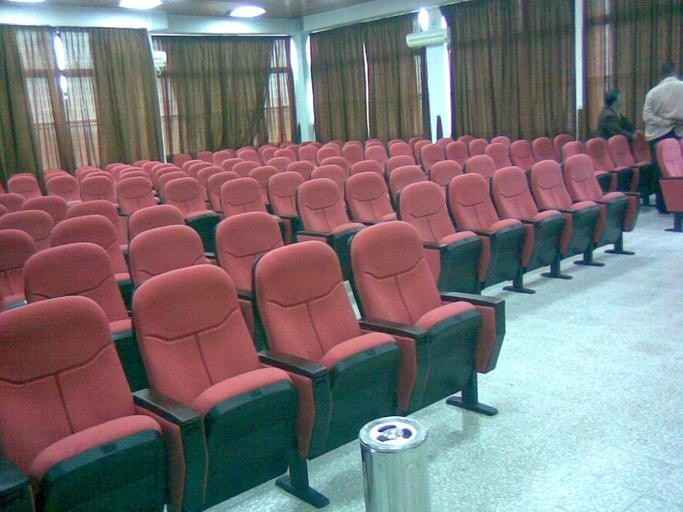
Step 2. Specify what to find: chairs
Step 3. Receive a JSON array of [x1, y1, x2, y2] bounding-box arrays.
[[0, 295, 165, 512], [130, 264, 299, 512], [250, 240, 431, 508], [346, 220, 505, 416]]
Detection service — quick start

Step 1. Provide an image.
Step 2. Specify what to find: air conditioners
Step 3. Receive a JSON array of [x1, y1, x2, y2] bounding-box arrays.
[[152, 50, 167, 69], [405, 29, 445, 47]]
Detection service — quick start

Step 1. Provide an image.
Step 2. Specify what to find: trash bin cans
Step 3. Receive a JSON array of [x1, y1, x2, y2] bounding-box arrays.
[[358, 415, 432, 512]]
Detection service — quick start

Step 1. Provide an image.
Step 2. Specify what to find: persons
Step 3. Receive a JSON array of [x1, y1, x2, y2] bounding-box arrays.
[[642, 62, 682, 215], [598, 88, 643, 144]]
